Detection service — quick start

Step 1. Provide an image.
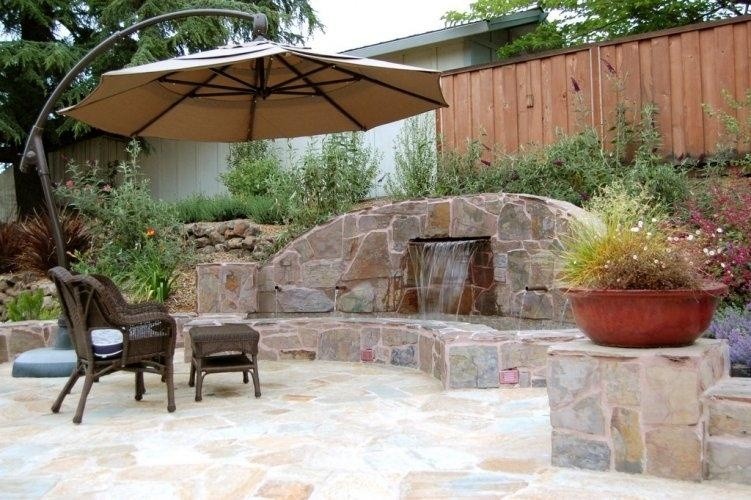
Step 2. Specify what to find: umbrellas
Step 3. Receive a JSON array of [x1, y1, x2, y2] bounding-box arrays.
[[18, 5, 454, 278]]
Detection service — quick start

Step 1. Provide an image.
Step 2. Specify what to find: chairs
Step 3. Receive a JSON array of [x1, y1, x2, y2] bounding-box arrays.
[[46, 266, 177, 424]]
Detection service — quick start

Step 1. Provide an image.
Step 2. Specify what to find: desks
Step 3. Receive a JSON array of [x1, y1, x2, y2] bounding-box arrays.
[[188, 324, 263, 401]]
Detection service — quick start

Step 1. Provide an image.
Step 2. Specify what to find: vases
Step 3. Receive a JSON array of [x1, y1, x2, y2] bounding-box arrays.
[[559, 282, 728, 348]]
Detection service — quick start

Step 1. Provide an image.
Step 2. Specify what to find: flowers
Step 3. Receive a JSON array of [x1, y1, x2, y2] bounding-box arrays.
[[548, 178, 728, 303]]
